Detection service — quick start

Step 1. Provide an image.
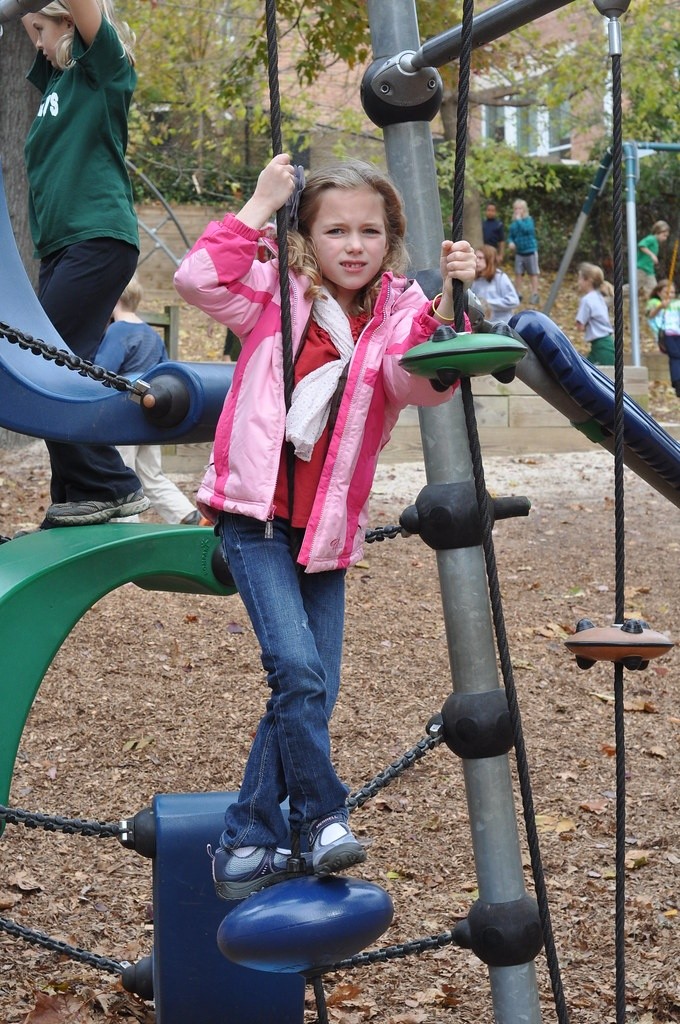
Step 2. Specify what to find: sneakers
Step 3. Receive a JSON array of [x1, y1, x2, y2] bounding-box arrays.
[[205, 842, 314, 900], [308, 813, 365, 877], [183, 509, 213, 526], [46, 484, 152, 525]]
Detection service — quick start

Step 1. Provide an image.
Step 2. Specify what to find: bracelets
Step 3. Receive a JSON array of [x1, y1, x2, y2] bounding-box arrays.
[[432, 291, 455, 321]]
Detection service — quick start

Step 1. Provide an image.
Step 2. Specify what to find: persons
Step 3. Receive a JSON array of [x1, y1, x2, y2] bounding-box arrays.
[[575, 263, 615, 365], [482, 203, 505, 262], [13, 0, 140, 540], [623, 220, 669, 298], [470, 245, 520, 325], [645, 279, 680, 398], [508, 199, 540, 302], [175, 153, 476, 899], [93, 277, 212, 525]]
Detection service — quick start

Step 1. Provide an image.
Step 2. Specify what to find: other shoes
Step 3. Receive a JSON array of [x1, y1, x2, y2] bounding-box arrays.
[[529, 294, 539, 304]]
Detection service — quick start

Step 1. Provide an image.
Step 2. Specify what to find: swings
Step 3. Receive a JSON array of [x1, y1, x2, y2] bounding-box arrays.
[[643, 236, 680, 353]]
